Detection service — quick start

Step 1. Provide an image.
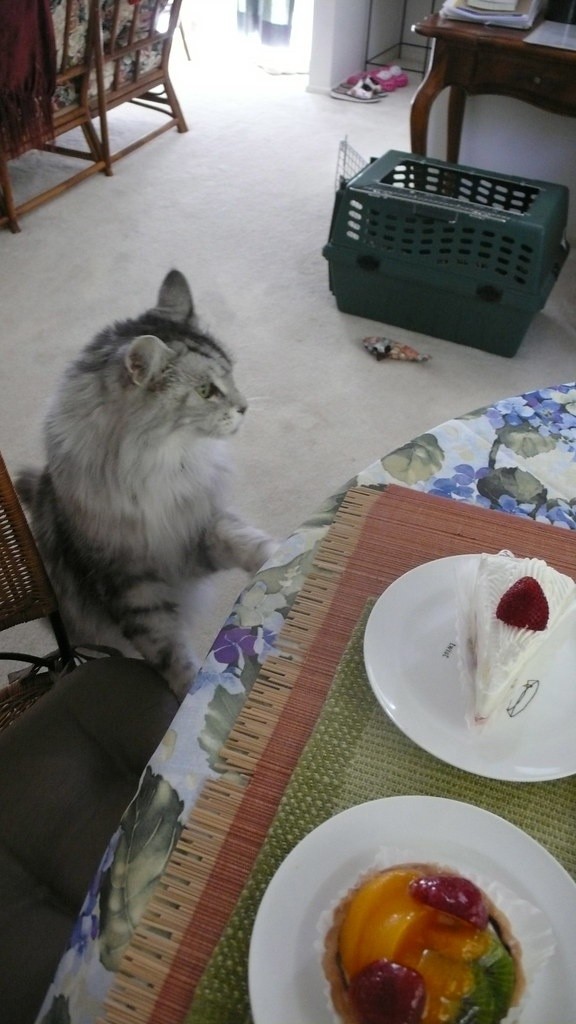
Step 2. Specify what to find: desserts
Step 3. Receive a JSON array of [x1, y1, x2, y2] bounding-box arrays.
[[332, 865, 521, 1023], [468, 551, 573, 719]]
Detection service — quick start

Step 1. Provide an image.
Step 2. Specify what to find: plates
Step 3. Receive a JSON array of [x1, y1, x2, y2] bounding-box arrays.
[[249, 795, 576, 1023], [361, 552, 576, 786]]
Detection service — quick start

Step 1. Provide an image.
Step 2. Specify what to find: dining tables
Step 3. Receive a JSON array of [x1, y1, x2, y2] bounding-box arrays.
[[30, 383, 576, 1024]]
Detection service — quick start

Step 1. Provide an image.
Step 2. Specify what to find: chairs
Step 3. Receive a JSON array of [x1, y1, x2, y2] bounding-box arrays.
[[0, 452, 184, 1023]]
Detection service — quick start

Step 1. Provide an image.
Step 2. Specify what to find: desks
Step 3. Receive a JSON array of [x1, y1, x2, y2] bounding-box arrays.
[[407, 10, 576, 194]]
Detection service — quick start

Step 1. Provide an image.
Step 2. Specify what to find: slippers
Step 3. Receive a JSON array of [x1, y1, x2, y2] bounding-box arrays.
[[331, 64, 410, 103]]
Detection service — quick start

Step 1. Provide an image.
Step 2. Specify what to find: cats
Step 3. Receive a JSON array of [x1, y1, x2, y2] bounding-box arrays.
[[14, 266, 281, 706]]
